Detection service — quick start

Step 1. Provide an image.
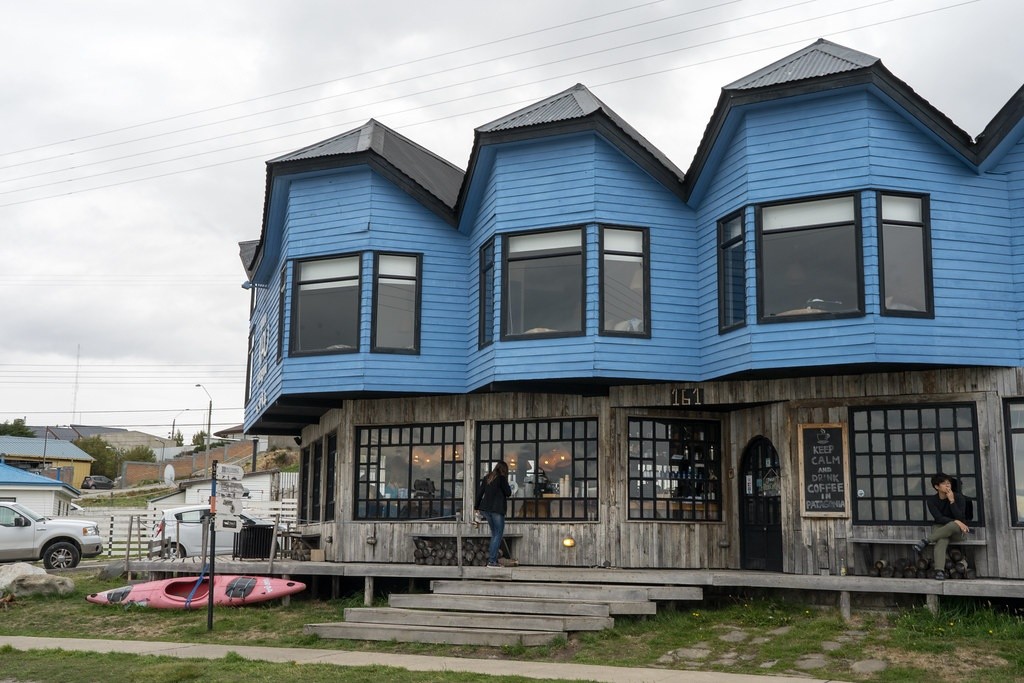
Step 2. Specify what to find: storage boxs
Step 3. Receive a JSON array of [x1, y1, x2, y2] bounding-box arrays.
[[310, 549, 325, 562]]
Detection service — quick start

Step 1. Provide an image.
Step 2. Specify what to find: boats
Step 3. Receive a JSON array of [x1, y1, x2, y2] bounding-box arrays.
[[85, 574, 305, 612]]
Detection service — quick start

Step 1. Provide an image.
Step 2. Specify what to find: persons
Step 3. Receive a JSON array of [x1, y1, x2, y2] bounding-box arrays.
[[913, 473, 973, 580], [475, 461, 511, 568]]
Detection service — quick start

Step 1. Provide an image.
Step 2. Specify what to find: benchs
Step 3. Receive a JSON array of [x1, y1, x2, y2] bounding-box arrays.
[[847, 537, 986, 579], [290, 533, 321, 559], [407, 532, 522, 566]]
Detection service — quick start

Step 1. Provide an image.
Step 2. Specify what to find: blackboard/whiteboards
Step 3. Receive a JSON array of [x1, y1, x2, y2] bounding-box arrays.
[[797, 423, 851, 518]]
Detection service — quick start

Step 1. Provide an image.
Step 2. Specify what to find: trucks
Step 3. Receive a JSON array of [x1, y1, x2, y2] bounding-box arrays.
[[215, 463, 245, 481]]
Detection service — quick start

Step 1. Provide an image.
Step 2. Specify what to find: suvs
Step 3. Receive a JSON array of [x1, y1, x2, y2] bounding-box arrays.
[[81, 476, 114, 491]]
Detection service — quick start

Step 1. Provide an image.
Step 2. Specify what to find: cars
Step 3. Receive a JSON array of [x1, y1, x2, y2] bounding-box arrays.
[[113, 476, 122, 486], [0, 501, 104, 569], [147, 505, 284, 559]]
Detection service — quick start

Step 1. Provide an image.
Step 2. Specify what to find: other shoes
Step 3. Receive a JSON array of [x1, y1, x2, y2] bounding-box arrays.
[[935, 570, 944, 580], [914, 538, 928, 552], [487, 561, 504, 568]]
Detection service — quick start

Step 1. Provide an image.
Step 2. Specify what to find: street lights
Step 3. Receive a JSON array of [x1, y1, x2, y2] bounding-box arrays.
[[154, 437, 165, 486], [195, 383, 212, 483], [172, 409, 190, 440]]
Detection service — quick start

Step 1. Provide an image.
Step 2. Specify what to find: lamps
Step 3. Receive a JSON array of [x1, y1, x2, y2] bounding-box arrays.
[[563, 538, 575, 547], [367, 537, 378, 544], [325, 536, 333, 542], [242, 281, 267, 288], [294, 437, 302, 446]]
